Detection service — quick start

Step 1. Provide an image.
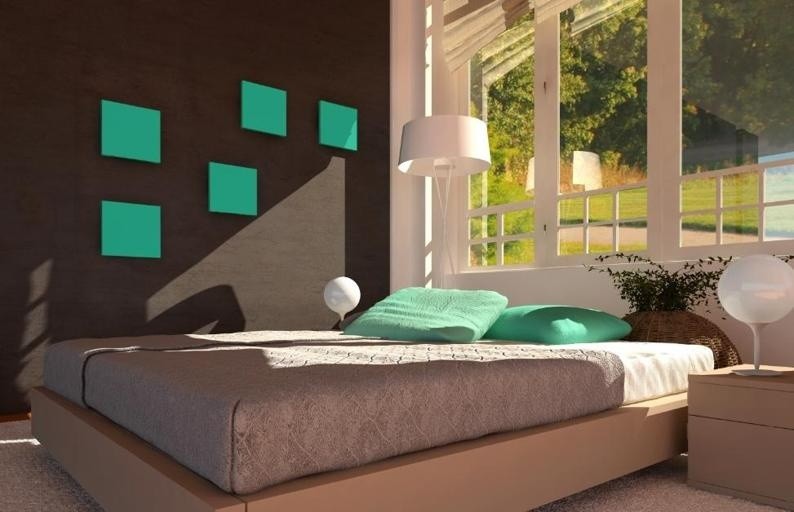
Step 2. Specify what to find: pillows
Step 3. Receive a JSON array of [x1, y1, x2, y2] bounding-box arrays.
[[483, 304, 630, 344], [337, 286, 504, 344], [336, 310, 369, 330]]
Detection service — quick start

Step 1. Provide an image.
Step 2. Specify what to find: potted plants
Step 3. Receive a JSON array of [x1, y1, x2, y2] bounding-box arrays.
[[582, 250, 793, 369]]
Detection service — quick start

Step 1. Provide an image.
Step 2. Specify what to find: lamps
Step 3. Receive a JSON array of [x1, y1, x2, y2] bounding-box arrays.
[[525, 150, 603, 193], [323, 276, 362, 321], [398, 113, 492, 286], [716, 253, 794, 377]]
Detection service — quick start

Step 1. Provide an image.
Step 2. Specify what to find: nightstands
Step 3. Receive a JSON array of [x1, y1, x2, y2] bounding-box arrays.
[[687, 363, 794, 510]]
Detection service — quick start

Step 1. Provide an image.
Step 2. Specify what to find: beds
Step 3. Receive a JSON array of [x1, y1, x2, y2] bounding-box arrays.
[[30, 285, 716, 512]]
